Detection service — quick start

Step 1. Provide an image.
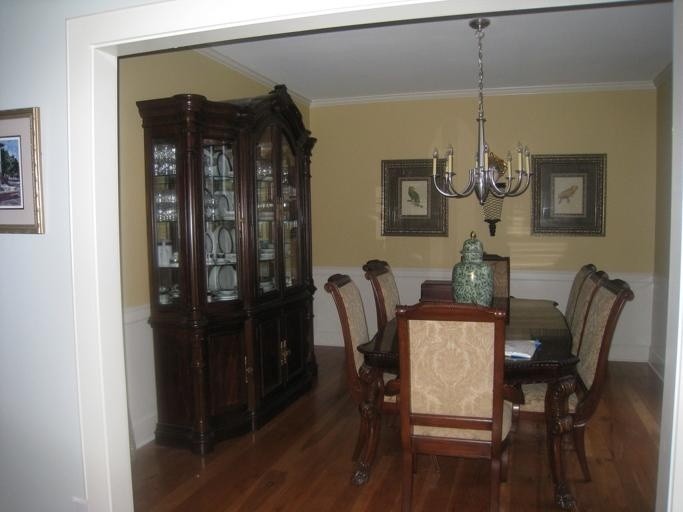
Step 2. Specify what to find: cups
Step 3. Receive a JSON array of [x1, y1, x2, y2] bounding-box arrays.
[[153, 142, 178, 222], [254, 157, 272, 182]]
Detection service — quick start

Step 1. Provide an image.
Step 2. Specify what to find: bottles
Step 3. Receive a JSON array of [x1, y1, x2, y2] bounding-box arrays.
[[282, 215, 297, 234]]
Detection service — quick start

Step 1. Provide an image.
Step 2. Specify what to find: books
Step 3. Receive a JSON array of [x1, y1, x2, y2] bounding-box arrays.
[[504, 339, 540, 359]]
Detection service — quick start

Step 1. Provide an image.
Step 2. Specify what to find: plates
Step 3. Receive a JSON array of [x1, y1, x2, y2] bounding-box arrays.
[[258, 209, 276, 222], [202, 146, 237, 298], [259, 244, 275, 260], [258, 280, 275, 293]]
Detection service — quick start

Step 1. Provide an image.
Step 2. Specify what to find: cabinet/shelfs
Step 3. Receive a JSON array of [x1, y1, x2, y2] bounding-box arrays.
[[134, 82, 319, 456]]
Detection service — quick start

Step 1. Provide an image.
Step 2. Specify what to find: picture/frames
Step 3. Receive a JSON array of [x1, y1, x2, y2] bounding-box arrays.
[[526, 151, 606, 235], [0, 106, 46, 236], [378, 156, 450, 239]]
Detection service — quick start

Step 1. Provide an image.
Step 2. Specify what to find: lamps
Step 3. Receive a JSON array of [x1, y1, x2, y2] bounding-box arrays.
[[429, 17, 532, 207], [472, 182, 507, 237]]
